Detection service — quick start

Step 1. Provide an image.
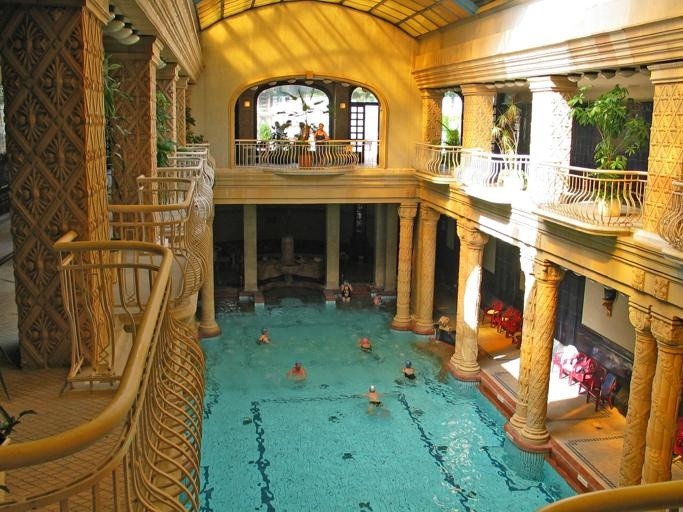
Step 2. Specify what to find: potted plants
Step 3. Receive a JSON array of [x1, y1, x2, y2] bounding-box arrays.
[[575, 84, 650, 218]]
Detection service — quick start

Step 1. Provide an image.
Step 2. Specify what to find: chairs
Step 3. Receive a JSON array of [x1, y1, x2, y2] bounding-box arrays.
[[554, 344, 616, 411], [482, 300, 522, 348]]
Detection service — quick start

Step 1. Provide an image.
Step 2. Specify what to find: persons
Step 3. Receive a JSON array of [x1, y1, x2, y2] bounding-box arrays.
[[286, 362, 305, 380], [272, 121, 282, 141], [340, 285, 353, 303], [371, 293, 382, 306], [293, 121, 306, 139], [400, 359, 417, 383], [437, 312, 449, 332], [312, 123, 331, 153], [254, 328, 270, 345], [359, 337, 374, 353], [361, 385, 389, 417]]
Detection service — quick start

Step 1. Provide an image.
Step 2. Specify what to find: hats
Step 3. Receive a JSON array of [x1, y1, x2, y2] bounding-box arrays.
[[362, 338, 411, 391], [262, 328, 268, 334]]
